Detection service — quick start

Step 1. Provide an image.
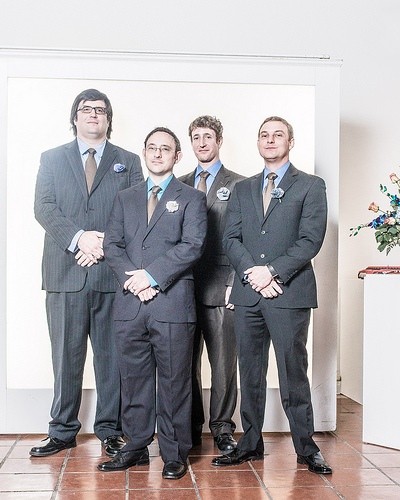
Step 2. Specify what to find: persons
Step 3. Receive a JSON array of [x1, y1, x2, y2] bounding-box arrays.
[[209, 116, 335, 477], [96, 126, 207, 479], [28, 89, 141, 458], [177, 115, 248, 451]]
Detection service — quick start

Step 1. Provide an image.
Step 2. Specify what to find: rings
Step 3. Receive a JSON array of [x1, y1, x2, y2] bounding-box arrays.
[[132, 286, 136, 292]]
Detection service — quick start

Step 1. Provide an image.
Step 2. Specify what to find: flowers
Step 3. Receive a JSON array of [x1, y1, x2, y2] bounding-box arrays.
[[114, 163, 124, 173], [216, 187, 231, 201], [166, 200, 180, 213], [349, 172, 400, 256], [271, 188, 284, 199]]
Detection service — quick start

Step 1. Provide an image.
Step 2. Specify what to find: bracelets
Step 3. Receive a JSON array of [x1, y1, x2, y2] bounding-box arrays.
[[264, 262, 279, 278]]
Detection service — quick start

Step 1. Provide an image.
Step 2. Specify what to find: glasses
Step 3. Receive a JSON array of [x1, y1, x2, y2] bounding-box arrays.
[[77, 106, 107, 115]]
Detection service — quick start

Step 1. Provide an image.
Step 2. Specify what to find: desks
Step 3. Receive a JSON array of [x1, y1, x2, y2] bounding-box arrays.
[[357, 265, 400, 451]]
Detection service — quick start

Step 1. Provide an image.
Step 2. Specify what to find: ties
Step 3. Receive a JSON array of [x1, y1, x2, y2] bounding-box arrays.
[[263, 173, 277, 214], [85, 148, 98, 194], [198, 171, 210, 195], [148, 186, 162, 225]]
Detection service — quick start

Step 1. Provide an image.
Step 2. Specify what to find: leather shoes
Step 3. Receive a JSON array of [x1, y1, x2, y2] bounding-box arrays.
[[215, 434, 238, 453], [162, 460, 187, 479], [98, 447, 150, 472], [101, 435, 127, 457], [296, 452, 333, 474], [29, 436, 77, 456], [211, 446, 264, 466]]
